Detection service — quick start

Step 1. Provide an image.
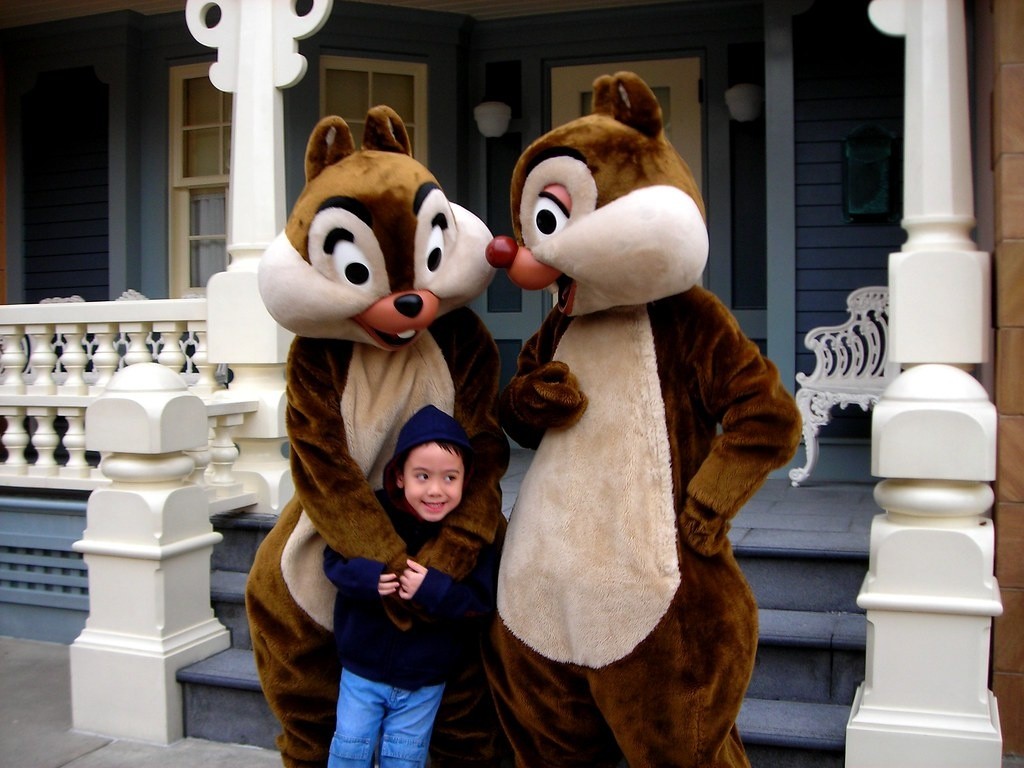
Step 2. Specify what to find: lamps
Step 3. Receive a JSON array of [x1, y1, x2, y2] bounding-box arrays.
[[724, 74, 766, 122], [472, 91, 513, 136]]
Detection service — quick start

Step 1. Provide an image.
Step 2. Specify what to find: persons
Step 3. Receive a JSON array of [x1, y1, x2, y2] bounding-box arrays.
[[321, 403, 502, 768]]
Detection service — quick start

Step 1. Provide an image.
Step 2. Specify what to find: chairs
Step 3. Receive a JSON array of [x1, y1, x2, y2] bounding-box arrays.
[[788, 285, 890, 487]]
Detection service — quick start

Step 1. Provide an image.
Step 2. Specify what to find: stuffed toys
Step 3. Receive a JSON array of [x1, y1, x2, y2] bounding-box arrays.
[[483, 69, 806, 768], [240, 102, 509, 766]]
